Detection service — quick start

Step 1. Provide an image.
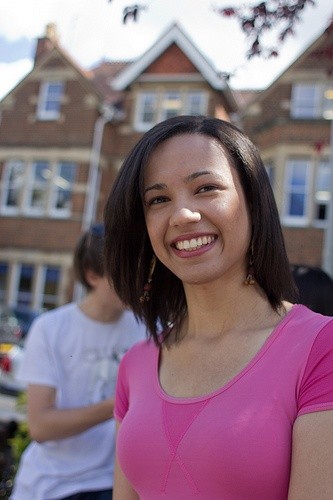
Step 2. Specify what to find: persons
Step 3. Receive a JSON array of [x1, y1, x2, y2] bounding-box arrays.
[[9, 223, 173, 500], [102, 114, 333, 500]]
